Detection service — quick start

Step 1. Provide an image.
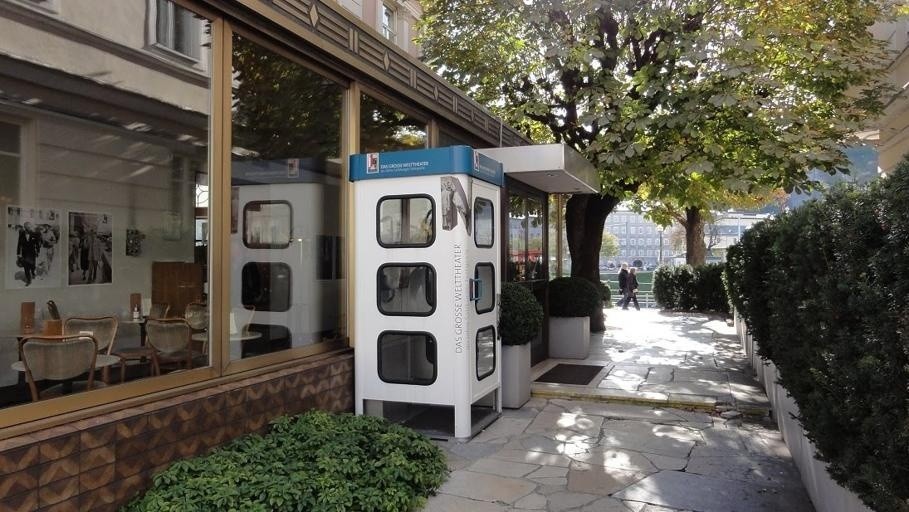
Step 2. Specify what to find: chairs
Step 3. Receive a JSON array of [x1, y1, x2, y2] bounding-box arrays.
[[1, 300, 257, 401]]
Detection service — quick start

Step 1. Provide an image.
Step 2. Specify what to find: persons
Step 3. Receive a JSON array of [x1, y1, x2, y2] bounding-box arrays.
[[622, 267, 641, 310], [615, 263, 631, 307], [16, 219, 104, 285]]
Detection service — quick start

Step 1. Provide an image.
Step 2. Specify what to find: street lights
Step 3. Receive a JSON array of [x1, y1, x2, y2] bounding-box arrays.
[[656, 226, 664, 268]]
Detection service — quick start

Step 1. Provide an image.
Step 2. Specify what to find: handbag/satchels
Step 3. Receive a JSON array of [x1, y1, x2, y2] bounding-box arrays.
[[628, 290, 634, 297]]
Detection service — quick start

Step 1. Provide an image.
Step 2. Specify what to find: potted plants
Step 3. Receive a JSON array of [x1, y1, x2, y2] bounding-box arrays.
[[496, 277, 598, 410]]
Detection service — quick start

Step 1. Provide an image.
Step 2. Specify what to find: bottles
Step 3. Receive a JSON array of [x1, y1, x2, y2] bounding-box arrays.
[[133, 304, 140, 319]]
